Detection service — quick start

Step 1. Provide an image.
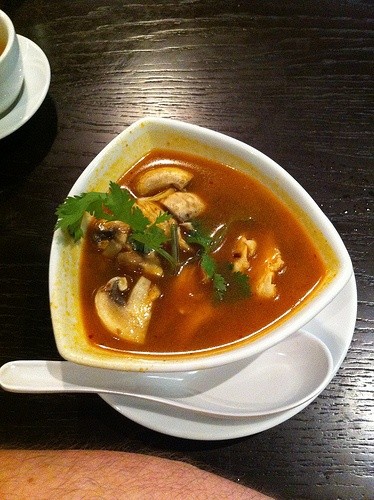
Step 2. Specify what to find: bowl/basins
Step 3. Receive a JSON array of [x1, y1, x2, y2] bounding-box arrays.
[[46, 114, 355, 372]]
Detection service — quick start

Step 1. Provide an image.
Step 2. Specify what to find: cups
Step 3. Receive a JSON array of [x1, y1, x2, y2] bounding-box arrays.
[[0, 9, 24, 119]]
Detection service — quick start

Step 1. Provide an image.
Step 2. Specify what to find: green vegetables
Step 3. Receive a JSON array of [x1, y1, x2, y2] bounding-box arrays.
[[54, 180, 257, 305]]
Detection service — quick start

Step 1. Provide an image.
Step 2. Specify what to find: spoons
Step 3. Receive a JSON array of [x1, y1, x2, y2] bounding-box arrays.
[[0, 332, 336, 418]]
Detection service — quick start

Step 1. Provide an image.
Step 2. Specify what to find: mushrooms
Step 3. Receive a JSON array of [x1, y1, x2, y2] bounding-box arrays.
[[93, 166, 284, 345]]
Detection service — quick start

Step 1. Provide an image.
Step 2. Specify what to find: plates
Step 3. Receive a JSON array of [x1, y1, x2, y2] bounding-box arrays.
[[95, 257, 356, 442], [0, 34, 50, 141]]
